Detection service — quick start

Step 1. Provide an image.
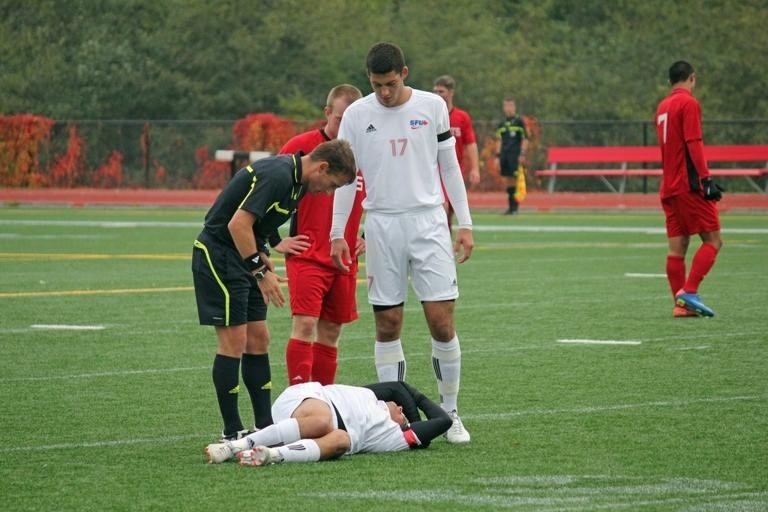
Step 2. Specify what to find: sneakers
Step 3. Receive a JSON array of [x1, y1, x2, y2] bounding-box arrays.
[[672, 288, 714, 318], [205, 425, 285, 467], [441, 406, 470, 444]]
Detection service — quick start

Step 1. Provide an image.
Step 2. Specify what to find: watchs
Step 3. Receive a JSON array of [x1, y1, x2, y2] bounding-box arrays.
[[253, 267, 270, 279]]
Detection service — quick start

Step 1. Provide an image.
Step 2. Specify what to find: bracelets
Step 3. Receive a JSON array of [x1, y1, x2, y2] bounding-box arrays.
[[266, 232, 282, 248], [241, 252, 265, 272]]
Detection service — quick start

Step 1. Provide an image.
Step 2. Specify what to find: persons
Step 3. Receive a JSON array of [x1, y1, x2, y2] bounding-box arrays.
[[327, 42, 474, 444], [432, 74, 481, 233], [653, 61, 724, 318], [191, 137, 356, 423], [494, 96, 530, 214], [278, 84, 366, 383], [205, 379, 453, 467]]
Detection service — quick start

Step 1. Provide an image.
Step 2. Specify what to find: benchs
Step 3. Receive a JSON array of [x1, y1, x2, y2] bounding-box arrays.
[[534, 145, 768, 194]]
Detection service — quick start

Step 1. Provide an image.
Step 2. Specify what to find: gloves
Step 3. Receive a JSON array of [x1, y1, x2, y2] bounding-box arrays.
[[702, 176, 724, 201]]
